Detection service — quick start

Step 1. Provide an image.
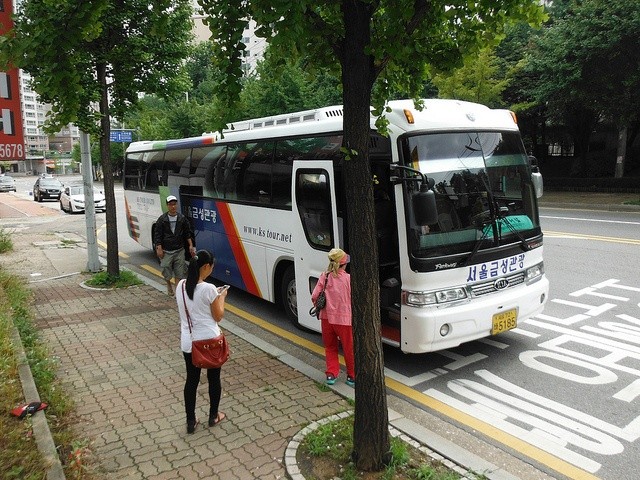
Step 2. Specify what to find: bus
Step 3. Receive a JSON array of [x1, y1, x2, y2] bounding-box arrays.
[[123, 99, 549, 354]]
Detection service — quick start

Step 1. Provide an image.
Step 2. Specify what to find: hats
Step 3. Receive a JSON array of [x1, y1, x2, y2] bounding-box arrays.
[[167, 195, 178, 202], [328, 249, 350, 277]]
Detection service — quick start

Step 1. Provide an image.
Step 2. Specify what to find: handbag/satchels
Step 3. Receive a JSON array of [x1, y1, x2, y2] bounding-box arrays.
[[309, 307, 320, 320], [317, 270, 333, 309], [182, 281, 229, 368]]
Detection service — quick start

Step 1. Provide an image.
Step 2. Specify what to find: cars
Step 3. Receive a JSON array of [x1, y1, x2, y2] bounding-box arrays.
[[60, 184, 106, 214], [0, 176, 16, 192], [33, 176, 64, 202]]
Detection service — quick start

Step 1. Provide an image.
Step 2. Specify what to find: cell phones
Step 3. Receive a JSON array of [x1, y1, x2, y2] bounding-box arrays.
[[218, 285, 230, 296]]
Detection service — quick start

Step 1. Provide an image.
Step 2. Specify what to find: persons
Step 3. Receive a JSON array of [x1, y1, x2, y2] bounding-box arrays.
[[154, 195, 194, 300], [311, 248, 356, 385], [460, 195, 485, 228], [175, 249, 228, 434]]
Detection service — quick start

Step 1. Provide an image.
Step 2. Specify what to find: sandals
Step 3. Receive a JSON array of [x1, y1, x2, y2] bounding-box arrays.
[[187, 418, 200, 433], [209, 411, 226, 427]]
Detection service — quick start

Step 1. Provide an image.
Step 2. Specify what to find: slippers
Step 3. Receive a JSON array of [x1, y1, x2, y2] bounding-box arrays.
[[168, 289, 174, 296]]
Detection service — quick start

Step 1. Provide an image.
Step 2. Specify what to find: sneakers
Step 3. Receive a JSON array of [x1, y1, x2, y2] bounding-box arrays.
[[327, 375, 340, 385], [345, 374, 355, 385]]
